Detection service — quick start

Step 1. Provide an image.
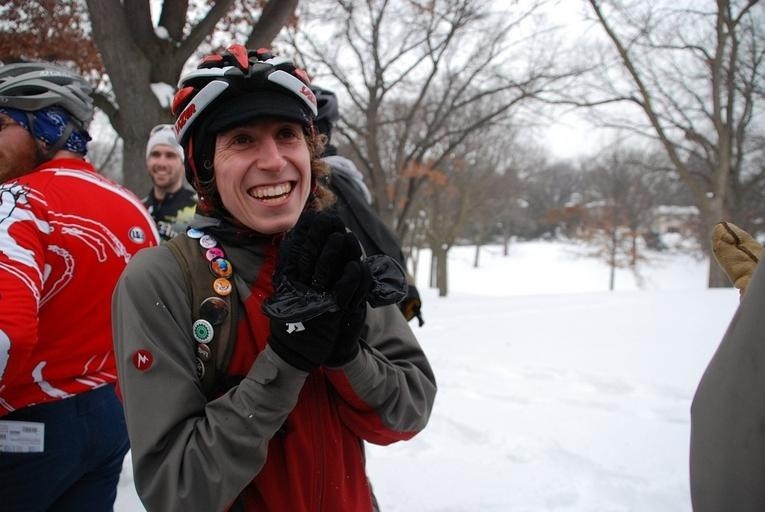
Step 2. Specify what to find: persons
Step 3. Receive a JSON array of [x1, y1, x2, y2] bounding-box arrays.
[[0, 62, 168, 512], [304, 82, 372, 215], [131, 117, 205, 258], [107, 43, 437, 511], [686, 218, 764, 512]]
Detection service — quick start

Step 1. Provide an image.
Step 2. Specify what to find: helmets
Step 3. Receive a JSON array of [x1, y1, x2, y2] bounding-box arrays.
[[309, 83, 340, 121], [142, 124, 183, 163], [171, 44, 319, 196], [1, 63, 94, 131]]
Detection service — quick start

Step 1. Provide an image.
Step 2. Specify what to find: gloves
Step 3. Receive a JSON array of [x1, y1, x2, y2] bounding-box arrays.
[[260, 206, 409, 372]]
